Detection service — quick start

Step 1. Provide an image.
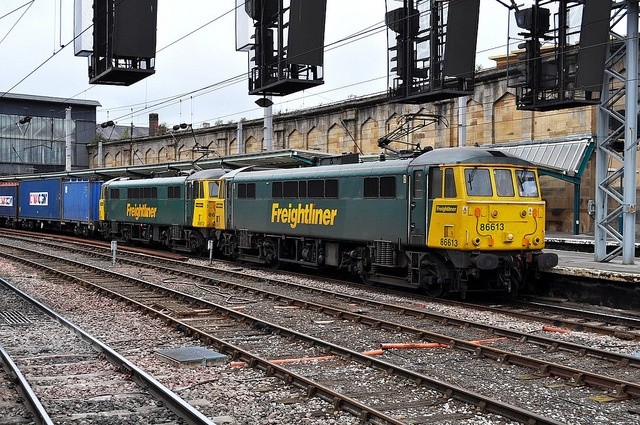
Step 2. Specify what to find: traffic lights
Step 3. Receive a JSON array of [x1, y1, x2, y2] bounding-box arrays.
[[517, 39, 542, 86], [514, 6, 550, 34], [384, 8, 419, 34], [243, 0, 282, 20], [389, 39, 414, 76], [248, 27, 273, 64]]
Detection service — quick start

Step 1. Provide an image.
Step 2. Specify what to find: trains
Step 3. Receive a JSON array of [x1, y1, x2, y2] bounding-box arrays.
[[0, 146, 558, 301]]
